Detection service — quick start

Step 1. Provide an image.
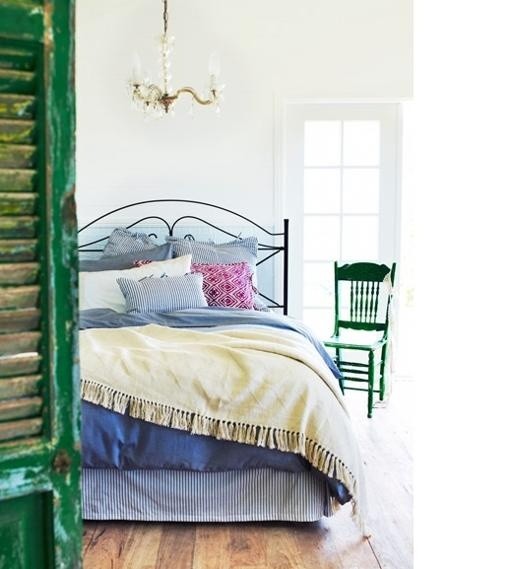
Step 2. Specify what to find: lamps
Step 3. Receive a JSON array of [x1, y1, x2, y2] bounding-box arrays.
[[126, 0, 220, 115]]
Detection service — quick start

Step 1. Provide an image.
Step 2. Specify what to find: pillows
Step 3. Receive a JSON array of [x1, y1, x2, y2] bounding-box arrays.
[[166, 235, 271, 312], [78, 241, 172, 272], [134, 259, 152, 268], [103, 228, 165, 254], [190, 261, 256, 311], [116, 271, 212, 315], [76, 253, 208, 311]]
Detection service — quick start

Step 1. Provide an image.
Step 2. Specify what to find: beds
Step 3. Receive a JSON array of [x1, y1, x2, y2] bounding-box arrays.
[[76, 199, 359, 526]]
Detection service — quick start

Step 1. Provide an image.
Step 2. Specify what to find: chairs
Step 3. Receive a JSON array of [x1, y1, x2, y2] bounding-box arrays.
[[322, 261, 396, 418]]
